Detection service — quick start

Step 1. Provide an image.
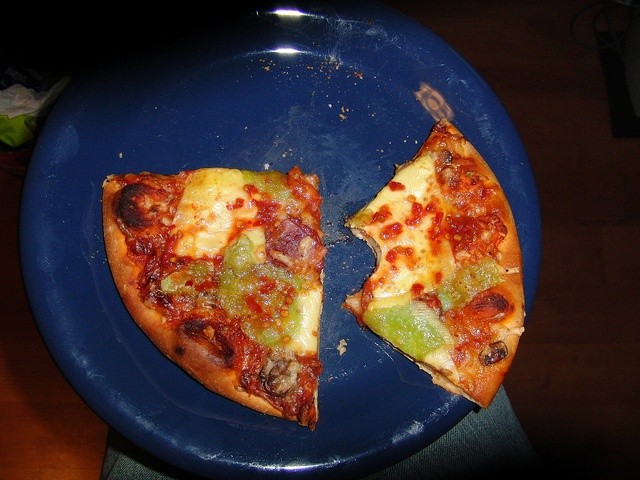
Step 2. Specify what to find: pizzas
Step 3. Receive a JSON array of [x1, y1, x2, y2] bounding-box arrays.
[[341, 118, 525, 408], [103, 167, 327, 433]]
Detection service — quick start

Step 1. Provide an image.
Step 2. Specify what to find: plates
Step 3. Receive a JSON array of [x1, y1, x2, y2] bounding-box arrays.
[[17, 1, 542, 477]]
